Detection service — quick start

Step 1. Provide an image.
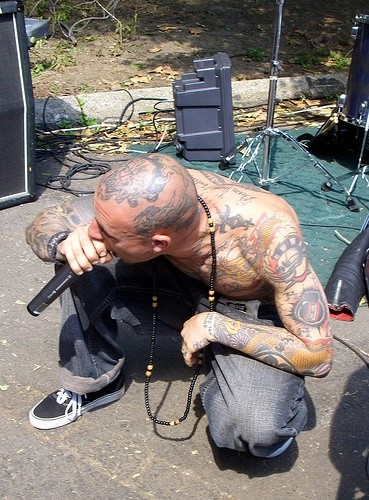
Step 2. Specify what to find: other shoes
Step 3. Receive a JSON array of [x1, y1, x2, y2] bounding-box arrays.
[[29, 369, 125, 430]]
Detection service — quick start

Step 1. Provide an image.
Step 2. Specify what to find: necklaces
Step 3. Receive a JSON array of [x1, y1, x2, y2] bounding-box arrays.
[[144, 194, 216, 426]]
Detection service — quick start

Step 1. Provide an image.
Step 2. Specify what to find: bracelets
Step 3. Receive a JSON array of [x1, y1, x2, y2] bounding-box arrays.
[[47, 231, 73, 266]]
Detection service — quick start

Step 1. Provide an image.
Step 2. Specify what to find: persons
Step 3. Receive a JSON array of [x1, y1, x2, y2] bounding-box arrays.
[[25, 152, 333, 458]]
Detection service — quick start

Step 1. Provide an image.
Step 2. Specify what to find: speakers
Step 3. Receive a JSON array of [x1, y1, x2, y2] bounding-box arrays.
[[0, 0, 38, 211], [172, 52, 236, 165]]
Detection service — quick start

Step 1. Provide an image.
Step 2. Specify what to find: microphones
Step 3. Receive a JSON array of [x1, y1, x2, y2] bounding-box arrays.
[[26, 262, 80, 316]]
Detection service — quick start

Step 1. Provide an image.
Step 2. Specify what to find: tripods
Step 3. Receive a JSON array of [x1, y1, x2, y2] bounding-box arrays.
[[218, 0, 359, 213]]
